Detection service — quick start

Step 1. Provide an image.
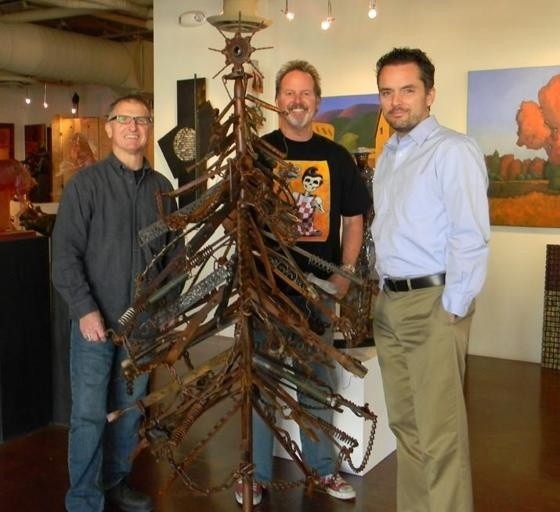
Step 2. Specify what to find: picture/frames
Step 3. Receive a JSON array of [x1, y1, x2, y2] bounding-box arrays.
[[0, 123, 15, 161]]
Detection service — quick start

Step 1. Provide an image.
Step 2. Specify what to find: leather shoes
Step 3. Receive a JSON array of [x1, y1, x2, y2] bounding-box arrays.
[[108, 480, 154, 511]]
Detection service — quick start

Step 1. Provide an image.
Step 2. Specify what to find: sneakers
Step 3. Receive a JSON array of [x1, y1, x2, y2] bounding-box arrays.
[[236, 477, 262, 505], [311, 473, 356, 500]]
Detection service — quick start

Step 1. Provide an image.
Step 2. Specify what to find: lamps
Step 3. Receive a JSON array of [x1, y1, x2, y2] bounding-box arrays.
[[280, 0, 293, 21], [320, 1, 336, 29], [24, 84, 78, 115], [368, 0, 377, 20]]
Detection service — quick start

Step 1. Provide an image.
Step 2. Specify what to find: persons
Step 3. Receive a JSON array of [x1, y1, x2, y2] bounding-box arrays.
[[220, 61, 369, 506], [370, 47, 491, 512], [51, 95, 187, 512]]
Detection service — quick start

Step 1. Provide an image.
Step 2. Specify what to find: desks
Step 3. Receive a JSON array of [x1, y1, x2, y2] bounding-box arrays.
[[0, 230, 53, 441]]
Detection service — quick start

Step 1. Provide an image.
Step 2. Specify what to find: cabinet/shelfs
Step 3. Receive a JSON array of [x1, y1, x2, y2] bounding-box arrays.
[[51, 115, 111, 202], [273, 345, 398, 479]]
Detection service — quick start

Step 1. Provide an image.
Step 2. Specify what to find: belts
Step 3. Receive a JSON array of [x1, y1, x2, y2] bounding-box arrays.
[[374, 273, 444, 292]]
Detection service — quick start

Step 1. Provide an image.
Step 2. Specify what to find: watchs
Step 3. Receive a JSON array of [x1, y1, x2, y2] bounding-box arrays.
[[341, 264, 356, 274]]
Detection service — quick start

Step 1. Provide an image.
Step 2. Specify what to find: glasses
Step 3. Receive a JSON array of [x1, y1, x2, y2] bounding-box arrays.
[[109, 115, 153, 126]]
